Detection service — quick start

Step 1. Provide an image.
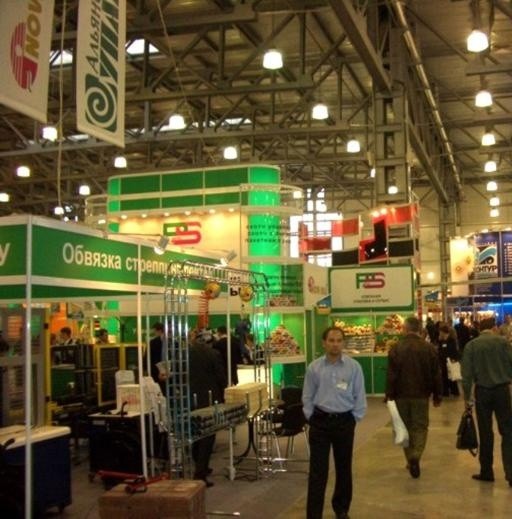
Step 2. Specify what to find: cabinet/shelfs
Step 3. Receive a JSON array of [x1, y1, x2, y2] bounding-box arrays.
[[96, 343, 147, 406], [328, 310, 415, 397], [241, 182, 307, 403]]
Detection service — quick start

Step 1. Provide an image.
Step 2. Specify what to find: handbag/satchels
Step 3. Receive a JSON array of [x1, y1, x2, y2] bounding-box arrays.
[[445, 356, 463, 381], [456, 413, 478, 449]]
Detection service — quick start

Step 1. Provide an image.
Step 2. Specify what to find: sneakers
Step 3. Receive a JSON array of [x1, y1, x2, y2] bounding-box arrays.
[[193, 468, 213, 487], [409, 458, 420, 478], [473, 474, 493, 481]]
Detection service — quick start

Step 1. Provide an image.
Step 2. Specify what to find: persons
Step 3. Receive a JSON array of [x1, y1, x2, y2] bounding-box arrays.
[[143, 323, 179, 416], [302, 327, 367, 518], [181, 329, 224, 487], [383, 318, 443, 478], [205, 326, 254, 402], [461, 312, 512, 486], [97, 329, 108, 344], [421, 314, 512, 407], [60, 328, 73, 345], [0, 331, 9, 352]]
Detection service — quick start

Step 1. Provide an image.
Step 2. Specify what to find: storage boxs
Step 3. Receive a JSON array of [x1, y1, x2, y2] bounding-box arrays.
[[99, 479, 207, 519]]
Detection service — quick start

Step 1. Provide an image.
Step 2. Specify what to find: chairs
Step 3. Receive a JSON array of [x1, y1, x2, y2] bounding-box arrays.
[[256, 388, 312, 475]]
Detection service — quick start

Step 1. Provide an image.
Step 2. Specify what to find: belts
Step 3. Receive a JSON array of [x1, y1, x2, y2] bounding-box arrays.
[[315, 408, 350, 418]]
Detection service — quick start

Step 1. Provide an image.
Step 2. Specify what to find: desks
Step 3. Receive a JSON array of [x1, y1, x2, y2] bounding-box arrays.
[[0, 425, 72, 519]]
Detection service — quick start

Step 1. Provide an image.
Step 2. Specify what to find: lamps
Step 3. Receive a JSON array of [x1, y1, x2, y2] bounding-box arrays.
[[221, 251, 238, 266], [467, 0, 490, 51], [482, 122, 496, 146], [168, 97, 186, 130], [154, 233, 171, 255], [484, 156, 500, 218], [223, 145, 237, 161], [311, 65, 329, 120], [388, 186, 398, 195], [474, 71, 493, 108], [0, 126, 128, 215], [346, 91, 362, 152], [263, 3, 283, 71], [370, 168, 377, 178]]
